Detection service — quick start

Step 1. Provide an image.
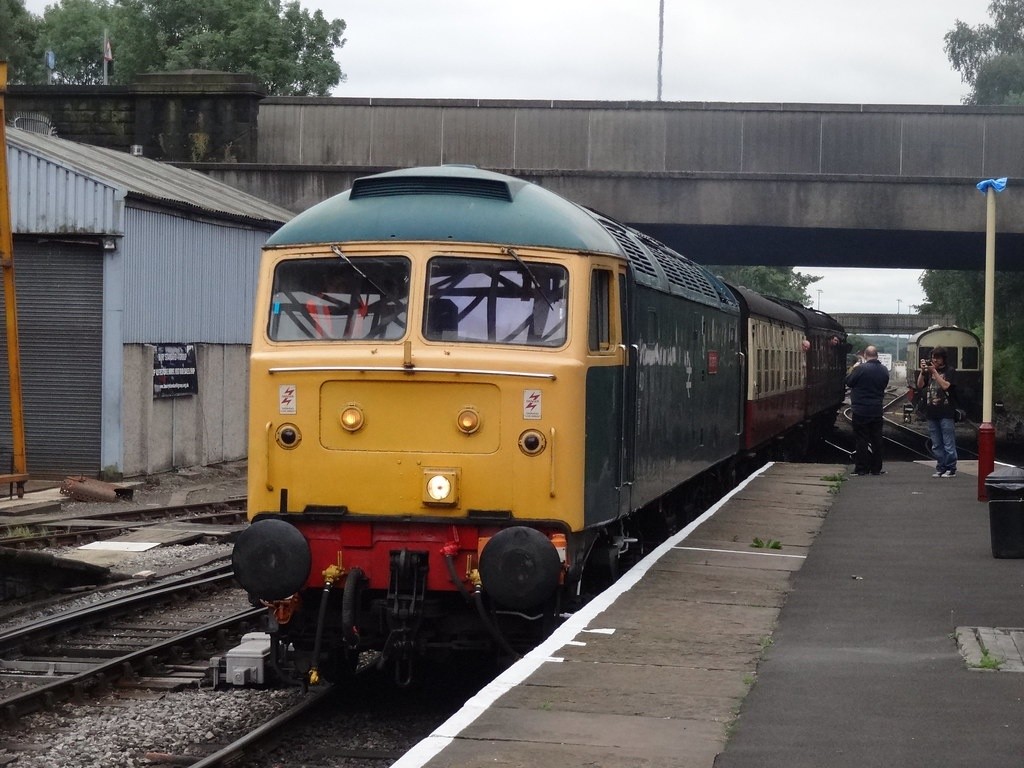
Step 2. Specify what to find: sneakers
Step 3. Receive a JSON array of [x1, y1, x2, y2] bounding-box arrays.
[[942, 469, 957, 478], [932, 472, 942, 477]]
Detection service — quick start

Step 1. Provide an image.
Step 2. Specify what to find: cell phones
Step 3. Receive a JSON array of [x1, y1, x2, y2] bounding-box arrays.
[[926, 360, 932, 367]]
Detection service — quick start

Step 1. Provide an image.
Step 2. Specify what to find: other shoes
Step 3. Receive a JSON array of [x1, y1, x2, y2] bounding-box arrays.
[[851, 472, 868, 477], [872, 471, 887, 477]]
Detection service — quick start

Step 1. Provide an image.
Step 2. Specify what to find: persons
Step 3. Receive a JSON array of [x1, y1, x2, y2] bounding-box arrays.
[[830, 334, 839, 346], [845, 345, 890, 476], [916, 345, 960, 478], [802, 340, 810, 353]]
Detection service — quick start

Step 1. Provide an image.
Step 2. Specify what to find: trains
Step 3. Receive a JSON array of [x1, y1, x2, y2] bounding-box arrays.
[[224, 153, 857, 679], [904, 322, 981, 407]]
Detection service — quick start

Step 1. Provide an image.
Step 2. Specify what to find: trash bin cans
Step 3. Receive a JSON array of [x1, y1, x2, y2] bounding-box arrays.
[[984, 466, 1024, 560]]
